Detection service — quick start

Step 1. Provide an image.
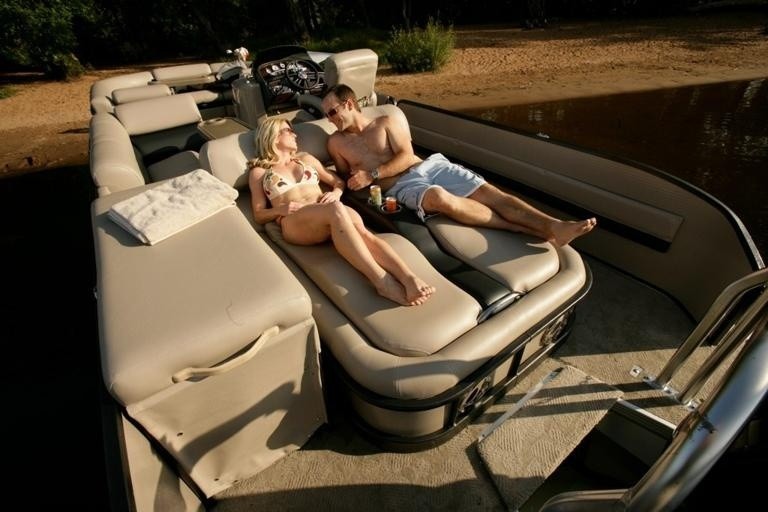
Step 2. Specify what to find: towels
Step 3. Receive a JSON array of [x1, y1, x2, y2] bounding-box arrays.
[[105, 167, 239, 247]]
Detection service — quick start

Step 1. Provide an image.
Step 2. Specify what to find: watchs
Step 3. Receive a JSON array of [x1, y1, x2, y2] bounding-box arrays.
[[368, 167, 380, 179]]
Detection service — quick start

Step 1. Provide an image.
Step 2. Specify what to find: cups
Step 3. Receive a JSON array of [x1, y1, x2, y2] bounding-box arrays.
[[386, 197, 397, 211]]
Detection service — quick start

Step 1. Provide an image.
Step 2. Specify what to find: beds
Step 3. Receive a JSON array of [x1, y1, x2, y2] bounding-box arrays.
[[201, 103, 593, 454]]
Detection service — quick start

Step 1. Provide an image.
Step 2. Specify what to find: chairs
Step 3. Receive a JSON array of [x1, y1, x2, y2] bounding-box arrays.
[[89, 93, 208, 196], [295, 48, 378, 120]]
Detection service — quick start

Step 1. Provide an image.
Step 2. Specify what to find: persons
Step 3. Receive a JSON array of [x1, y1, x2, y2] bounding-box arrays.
[[249, 118, 436, 307], [321, 84, 597, 248]]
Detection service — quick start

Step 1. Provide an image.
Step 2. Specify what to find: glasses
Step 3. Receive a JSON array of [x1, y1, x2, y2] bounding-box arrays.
[[324, 104, 343, 118]]
